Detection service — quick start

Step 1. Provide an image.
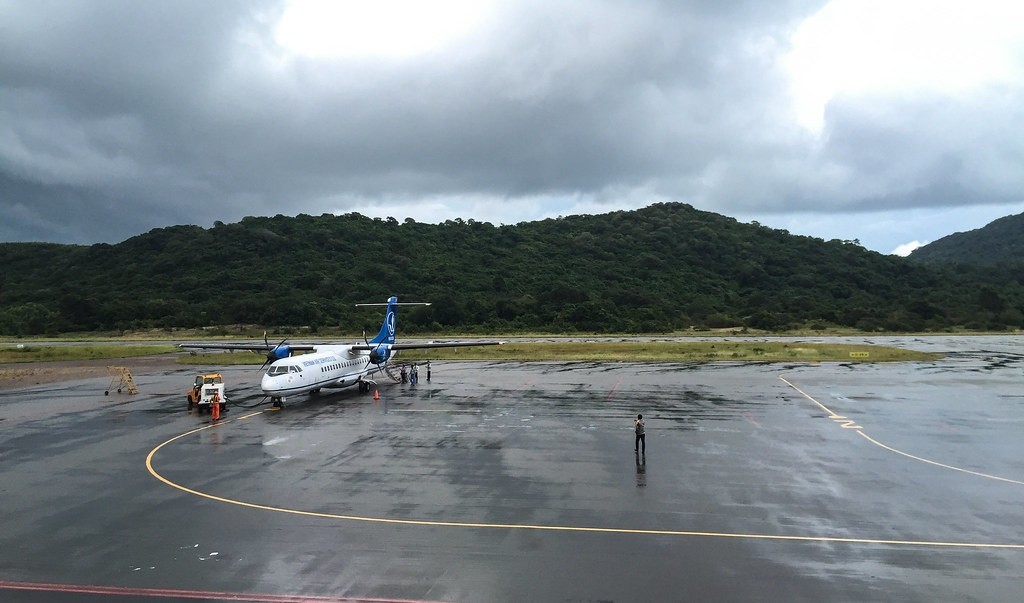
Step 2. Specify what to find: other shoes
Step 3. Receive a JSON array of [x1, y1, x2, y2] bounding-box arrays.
[[634, 448, 638, 452]]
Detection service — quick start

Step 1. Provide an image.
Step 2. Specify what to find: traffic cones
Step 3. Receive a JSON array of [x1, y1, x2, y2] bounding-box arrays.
[[373, 389, 379, 400]]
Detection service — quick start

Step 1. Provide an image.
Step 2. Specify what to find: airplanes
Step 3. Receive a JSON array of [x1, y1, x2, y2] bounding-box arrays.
[[178, 296, 509, 409]]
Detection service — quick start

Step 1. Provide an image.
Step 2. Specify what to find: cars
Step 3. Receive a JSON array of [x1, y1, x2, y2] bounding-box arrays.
[[187, 373, 226, 413]]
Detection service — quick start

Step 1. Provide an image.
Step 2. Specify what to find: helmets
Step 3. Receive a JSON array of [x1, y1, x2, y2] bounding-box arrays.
[[214, 391, 218, 395]]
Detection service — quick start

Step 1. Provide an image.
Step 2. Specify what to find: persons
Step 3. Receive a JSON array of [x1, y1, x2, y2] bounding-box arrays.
[[210, 391, 221, 421], [634, 414, 646, 454], [398, 363, 408, 384], [425, 361, 432, 381], [413, 363, 419, 383], [408, 364, 416, 386]]
